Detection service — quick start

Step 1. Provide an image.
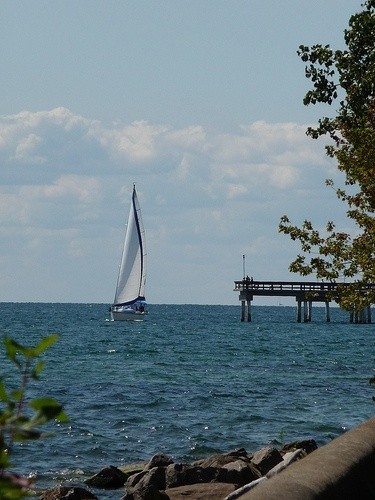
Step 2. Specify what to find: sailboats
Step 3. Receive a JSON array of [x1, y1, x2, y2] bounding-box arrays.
[[109, 180, 148, 321]]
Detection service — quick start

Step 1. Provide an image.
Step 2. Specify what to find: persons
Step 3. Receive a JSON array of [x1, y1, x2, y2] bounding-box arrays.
[[241, 274, 255, 289], [138, 303, 145, 313]]
